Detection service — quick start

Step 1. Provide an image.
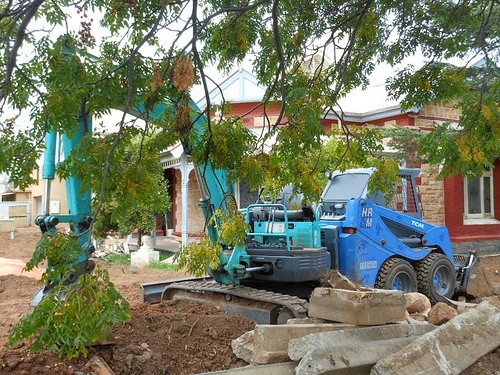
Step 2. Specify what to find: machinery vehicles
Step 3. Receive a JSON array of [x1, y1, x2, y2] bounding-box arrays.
[[26, 36, 457, 349]]
